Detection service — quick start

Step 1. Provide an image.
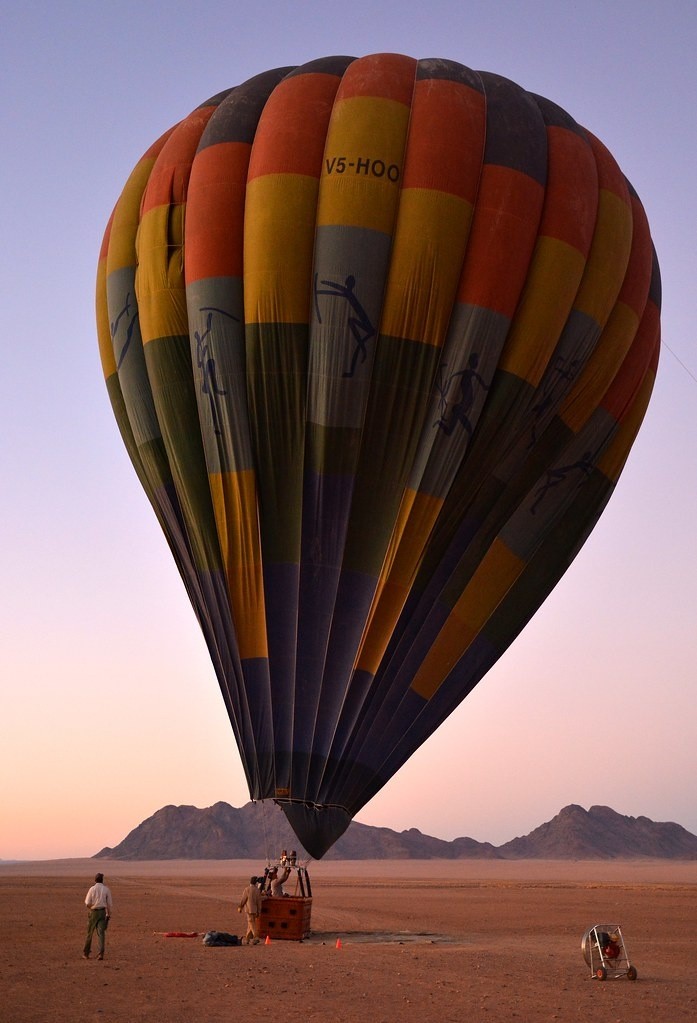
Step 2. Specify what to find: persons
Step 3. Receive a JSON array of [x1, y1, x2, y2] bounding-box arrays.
[[80, 873, 113, 960], [237, 866, 291, 943]]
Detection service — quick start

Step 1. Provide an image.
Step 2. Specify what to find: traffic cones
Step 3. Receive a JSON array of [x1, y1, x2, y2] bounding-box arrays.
[[264, 935, 273, 945], [335, 937, 343, 949]]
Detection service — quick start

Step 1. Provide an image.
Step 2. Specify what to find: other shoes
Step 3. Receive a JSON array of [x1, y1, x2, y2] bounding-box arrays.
[[82, 955, 89, 959], [247, 939, 250, 943], [97, 955, 103, 960], [253, 940, 260, 945]]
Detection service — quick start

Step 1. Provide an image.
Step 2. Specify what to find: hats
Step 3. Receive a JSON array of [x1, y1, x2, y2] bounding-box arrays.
[[95, 873, 104, 882]]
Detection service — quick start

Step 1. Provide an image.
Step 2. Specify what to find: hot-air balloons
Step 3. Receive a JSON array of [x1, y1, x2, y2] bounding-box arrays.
[[90, 52, 663, 939]]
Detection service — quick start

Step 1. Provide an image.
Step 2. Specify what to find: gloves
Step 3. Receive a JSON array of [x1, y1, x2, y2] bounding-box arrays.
[[238, 908, 242, 913]]
